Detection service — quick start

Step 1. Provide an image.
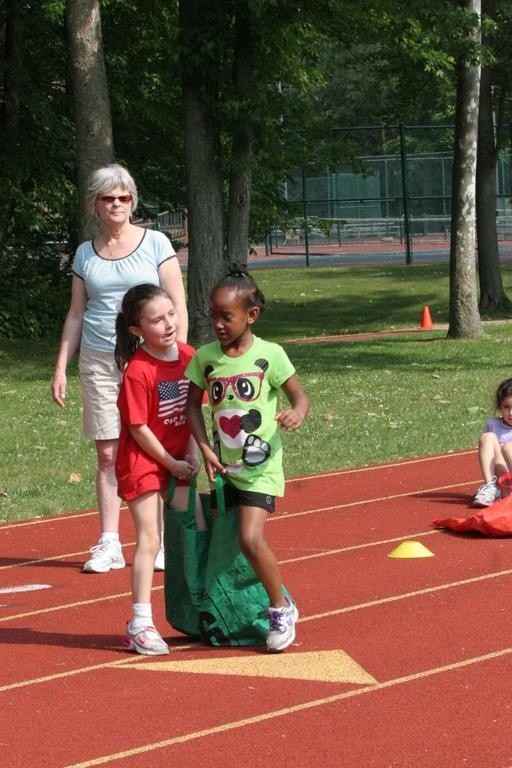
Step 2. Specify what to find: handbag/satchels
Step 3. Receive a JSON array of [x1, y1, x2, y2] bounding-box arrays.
[[163, 474, 270, 646]]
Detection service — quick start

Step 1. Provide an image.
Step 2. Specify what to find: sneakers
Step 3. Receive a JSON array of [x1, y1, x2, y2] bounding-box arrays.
[[84, 542, 125, 571], [474, 482, 503, 505], [265, 595, 299, 652], [123, 620, 169, 656]]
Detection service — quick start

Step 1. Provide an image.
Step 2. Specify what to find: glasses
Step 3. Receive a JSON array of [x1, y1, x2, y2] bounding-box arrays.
[[96, 195, 134, 203]]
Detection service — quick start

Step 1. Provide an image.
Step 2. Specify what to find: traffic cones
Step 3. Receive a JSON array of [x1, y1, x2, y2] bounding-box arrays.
[[418, 304, 433, 330]]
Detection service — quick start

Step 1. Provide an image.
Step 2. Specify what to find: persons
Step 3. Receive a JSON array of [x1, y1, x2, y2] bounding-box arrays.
[[114, 284, 209, 656], [182, 260, 311, 652], [50, 163, 190, 574], [470, 379, 512, 508]]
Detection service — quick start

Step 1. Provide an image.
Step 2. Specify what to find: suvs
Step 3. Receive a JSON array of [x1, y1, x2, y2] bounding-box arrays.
[[6, 210, 71, 255]]
